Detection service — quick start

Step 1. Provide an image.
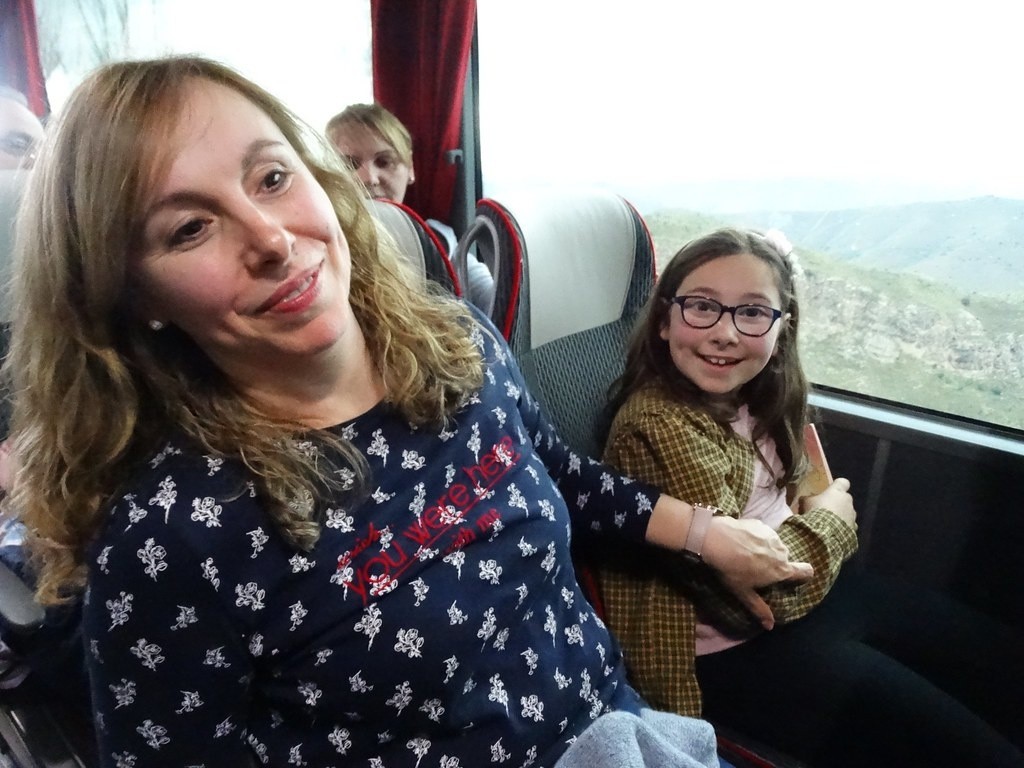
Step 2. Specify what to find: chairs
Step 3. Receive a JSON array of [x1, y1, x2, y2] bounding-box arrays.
[[451, 189, 660, 621]]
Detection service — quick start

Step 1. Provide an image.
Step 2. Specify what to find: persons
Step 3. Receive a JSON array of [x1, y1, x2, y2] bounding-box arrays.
[[0, 56, 815, 768], [587, 226, 1022, 768], [320, 101, 494, 317], [0, 85, 46, 170]]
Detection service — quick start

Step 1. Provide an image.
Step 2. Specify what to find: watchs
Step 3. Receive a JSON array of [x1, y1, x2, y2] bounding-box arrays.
[[680, 501, 725, 563]]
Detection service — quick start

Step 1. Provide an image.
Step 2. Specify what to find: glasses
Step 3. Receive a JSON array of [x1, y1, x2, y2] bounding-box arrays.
[[668, 295, 785, 337]]
